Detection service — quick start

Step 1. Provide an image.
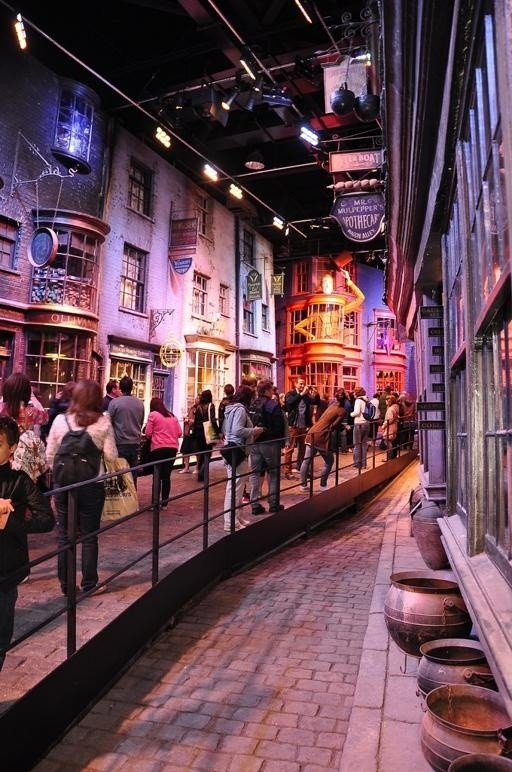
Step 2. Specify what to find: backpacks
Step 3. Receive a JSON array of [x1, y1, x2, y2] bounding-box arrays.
[[360, 397, 377, 422], [52, 415, 105, 489]]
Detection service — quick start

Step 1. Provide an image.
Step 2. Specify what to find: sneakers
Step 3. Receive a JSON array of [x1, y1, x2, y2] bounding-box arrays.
[[85, 582, 105, 596], [270, 504, 284, 512], [252, 505, 266, 515], [224, 518, 250, 531]]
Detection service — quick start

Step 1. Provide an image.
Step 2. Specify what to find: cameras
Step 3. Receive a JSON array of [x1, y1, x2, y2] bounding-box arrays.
[[257, 422, 269, 434], [307, 385, 313, 390]]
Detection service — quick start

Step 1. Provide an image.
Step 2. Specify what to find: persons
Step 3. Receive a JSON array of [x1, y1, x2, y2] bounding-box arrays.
[[293, 267, 366, 343], [2, 373, 422, 681]]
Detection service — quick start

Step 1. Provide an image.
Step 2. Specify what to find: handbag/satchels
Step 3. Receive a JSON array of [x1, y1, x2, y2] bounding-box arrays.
[[219, 441, 246, 467], [203, 421, 223, 444], [11, 429, 51, 482]]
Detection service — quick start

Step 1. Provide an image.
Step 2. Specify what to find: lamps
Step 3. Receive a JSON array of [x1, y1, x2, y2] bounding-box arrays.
[[220, 56, 264, 112], [309, 215, 330, 229], [244, 148, 265, 170], [272, 215, 292, 238], [298, 124, 321, 146], [147, 307, 183, 367], [201, 162, 219, 182], [228, 183, 243, 200], [153, 126, 172, 148]]
